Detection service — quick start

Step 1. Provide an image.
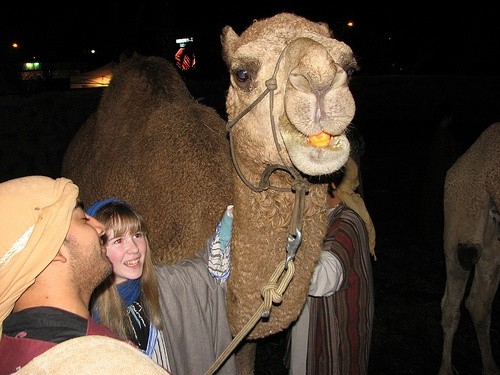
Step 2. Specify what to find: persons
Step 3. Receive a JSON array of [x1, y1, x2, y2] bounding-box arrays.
[[284, 159, 374, 375], [0, 175, 169, 375], [87, 198, 236, 375]]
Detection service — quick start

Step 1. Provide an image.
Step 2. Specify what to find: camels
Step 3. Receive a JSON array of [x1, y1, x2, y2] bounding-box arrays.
[[58, 11, 359, 375], [438, 120, 500, 375]]
